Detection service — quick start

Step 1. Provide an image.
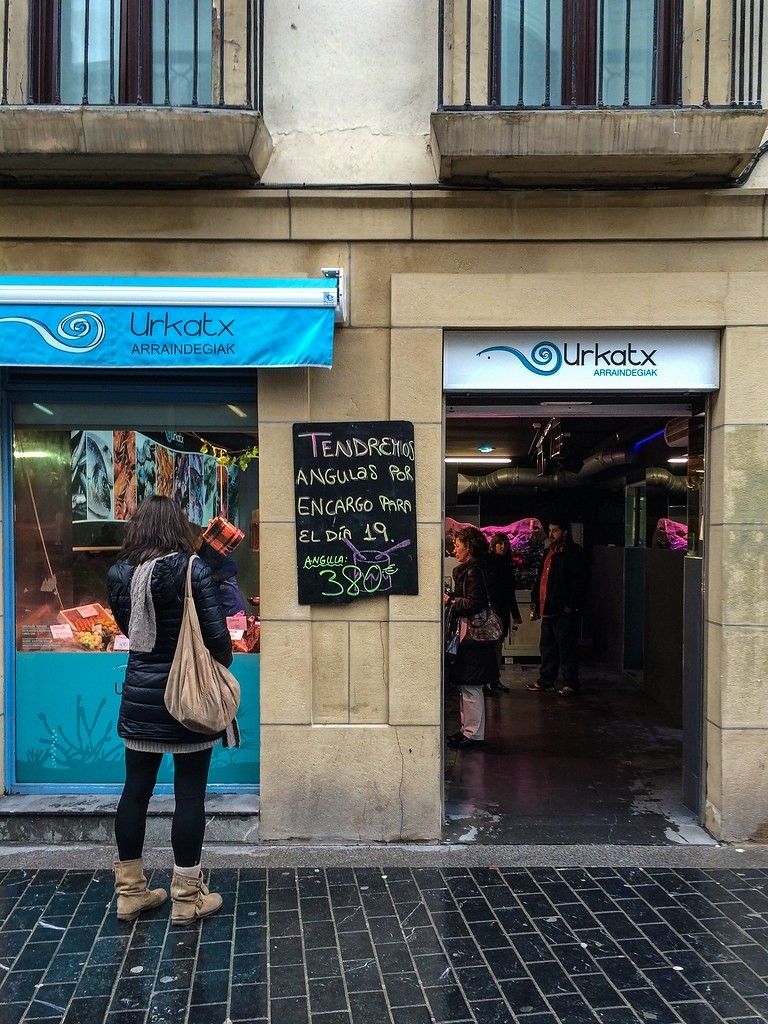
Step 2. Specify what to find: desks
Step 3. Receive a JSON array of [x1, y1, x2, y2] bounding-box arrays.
[[502, 590, 542, 664]]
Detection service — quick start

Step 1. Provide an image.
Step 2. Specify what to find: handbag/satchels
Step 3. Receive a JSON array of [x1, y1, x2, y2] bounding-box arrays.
[[455, 567, 503, 641], [442, 598, 460, 654], [164, 555, 240, 733]]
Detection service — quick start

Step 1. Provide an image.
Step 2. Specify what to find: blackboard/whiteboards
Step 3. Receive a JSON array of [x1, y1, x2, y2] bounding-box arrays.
[[290, 420, 418, 605]]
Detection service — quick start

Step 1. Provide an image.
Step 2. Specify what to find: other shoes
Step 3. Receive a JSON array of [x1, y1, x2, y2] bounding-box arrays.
[[482, 683, 500, 695], [489, 680, 510, 691], [446, 730, 481, 749]]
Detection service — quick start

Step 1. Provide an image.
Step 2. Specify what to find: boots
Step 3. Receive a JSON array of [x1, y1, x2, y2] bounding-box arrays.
[[113, 856, 168, 921], [170, 869, 222, 926]]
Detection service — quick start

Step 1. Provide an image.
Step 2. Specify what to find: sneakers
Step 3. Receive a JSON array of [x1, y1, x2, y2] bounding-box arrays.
[[525, 681, 554, 691], [558, 684, 581, 696]]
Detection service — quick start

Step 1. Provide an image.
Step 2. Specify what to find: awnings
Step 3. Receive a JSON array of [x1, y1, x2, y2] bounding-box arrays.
[[0, 267, 345, 370]]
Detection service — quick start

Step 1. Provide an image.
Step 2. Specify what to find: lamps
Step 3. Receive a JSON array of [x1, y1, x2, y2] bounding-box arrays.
[[478, 444, 495, 454], [668, 455, 688, 464]]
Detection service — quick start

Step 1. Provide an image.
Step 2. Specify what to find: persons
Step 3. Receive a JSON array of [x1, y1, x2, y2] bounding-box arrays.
[[443, 525, 499, 749], [484, 532, 523, 697], [107, 496, 234, 927], [189, 521, 247, 617], [526, 519, 592, 695]]
